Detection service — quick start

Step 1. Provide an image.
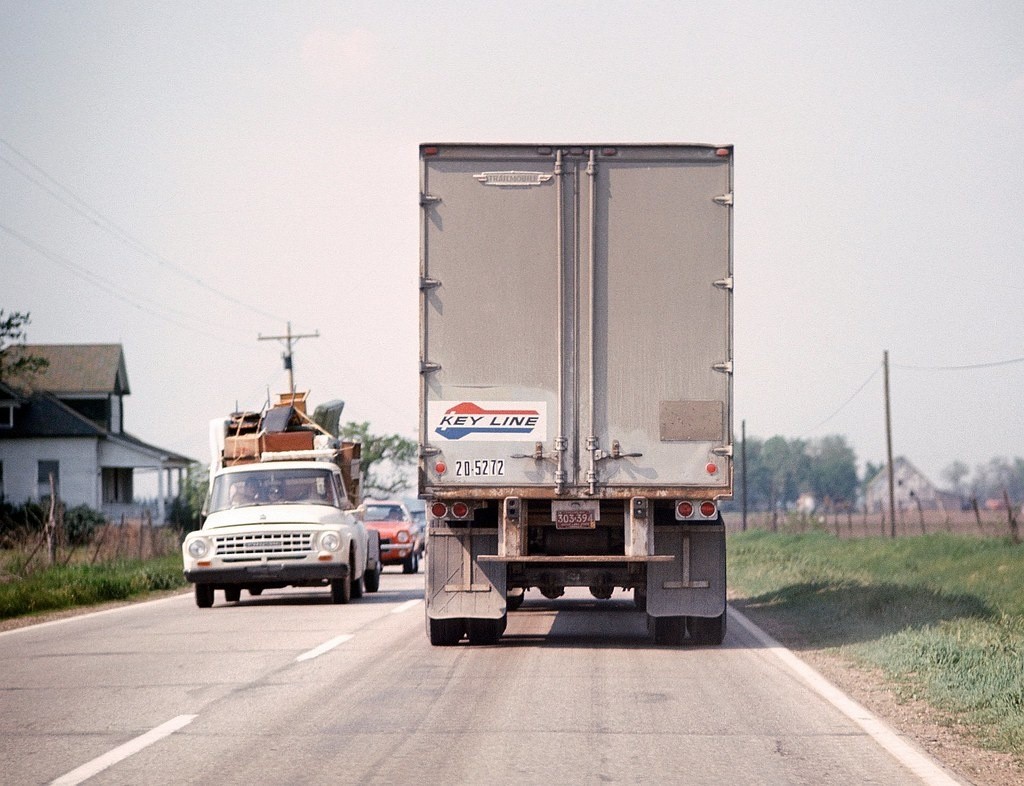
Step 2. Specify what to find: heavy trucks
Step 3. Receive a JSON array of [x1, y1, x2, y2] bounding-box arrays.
[[181, 461, 380, 608]]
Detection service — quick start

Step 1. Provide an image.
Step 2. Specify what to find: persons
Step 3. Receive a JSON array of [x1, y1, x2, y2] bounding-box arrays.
[[233, 477, 284, 505], [384, 509, 402, 521]]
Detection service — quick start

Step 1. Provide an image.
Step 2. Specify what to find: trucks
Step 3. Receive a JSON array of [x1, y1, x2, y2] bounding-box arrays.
[[417, 142, 735, 647]]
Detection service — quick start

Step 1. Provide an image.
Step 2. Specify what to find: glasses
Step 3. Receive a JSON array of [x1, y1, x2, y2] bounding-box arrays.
[[269, 489, 279, 493]]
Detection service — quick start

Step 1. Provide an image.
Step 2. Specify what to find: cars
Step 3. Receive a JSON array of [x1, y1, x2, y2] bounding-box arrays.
[[362, 499, 423, 575]]
